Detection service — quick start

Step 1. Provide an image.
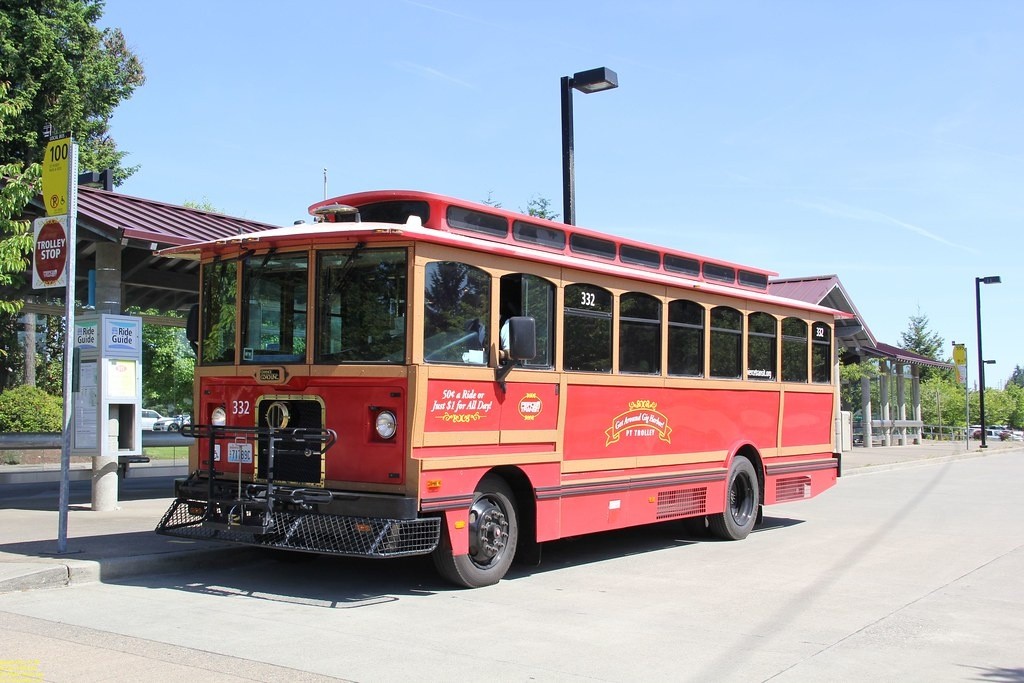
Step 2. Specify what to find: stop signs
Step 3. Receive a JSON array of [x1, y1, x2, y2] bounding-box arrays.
[[33, 211, 68, 292]]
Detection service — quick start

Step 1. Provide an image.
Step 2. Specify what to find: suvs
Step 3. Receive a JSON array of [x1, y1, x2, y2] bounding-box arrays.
[[142, 408, 163, 431]]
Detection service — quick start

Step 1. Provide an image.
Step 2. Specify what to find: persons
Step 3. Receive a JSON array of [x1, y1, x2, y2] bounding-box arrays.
[[461, 291, 515, 364]]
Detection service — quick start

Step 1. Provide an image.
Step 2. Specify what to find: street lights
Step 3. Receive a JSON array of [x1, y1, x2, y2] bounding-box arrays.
[[975, 275, 1001, 448], [559, 66, 619, 230], [982, 360, 996, 391]]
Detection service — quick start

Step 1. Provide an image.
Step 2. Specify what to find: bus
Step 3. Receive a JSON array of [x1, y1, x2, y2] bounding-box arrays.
[[151, 189, 856, 588]]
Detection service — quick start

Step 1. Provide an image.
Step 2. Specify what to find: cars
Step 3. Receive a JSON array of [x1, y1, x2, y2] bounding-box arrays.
[[971, 425, 1024, 443], [153, 414, 191, 432]]
